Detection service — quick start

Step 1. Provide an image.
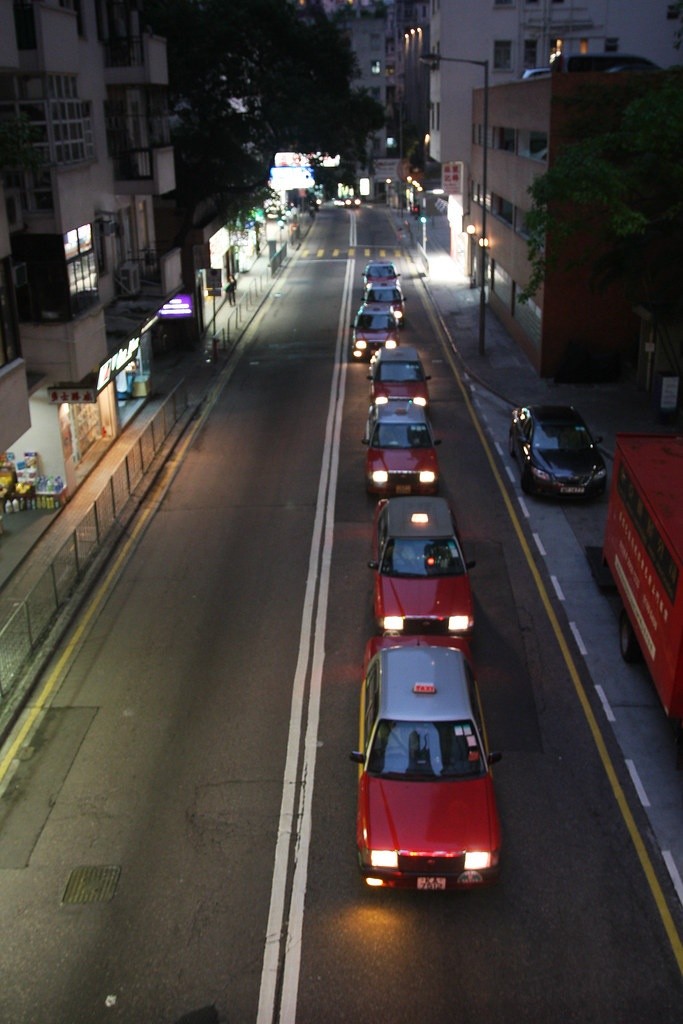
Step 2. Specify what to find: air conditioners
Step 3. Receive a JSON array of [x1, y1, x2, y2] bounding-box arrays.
[[14, 261, 30, 287], [119, 262, 141, 295], [5, 187, 25, 233]]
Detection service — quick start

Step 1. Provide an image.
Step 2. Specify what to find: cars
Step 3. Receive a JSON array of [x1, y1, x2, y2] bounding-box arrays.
[[350, 310, 402, 364], [361, 281, 407, 328], [519, 66, 551, 80], [361, 401, 441, 497], [367, 496, 478, 638], [366, 345, 432, 414], [509, 404, 606, 504], [361, 260, 400, 288], [347, 637, 505, 893]]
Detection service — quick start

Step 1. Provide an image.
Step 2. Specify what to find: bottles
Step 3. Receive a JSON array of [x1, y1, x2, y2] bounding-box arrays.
[[5, 473, 64, 513]]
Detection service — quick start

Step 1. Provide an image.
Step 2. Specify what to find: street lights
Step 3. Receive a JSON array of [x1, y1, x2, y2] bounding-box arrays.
[[419, 53, 489, 359]]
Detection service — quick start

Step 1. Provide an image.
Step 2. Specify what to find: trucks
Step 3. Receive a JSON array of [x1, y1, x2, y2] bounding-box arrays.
[[601, 429, 683, 721]]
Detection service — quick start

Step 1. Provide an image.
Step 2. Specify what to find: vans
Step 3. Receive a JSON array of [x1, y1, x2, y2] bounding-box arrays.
[[551, 52, 664, 74]]
